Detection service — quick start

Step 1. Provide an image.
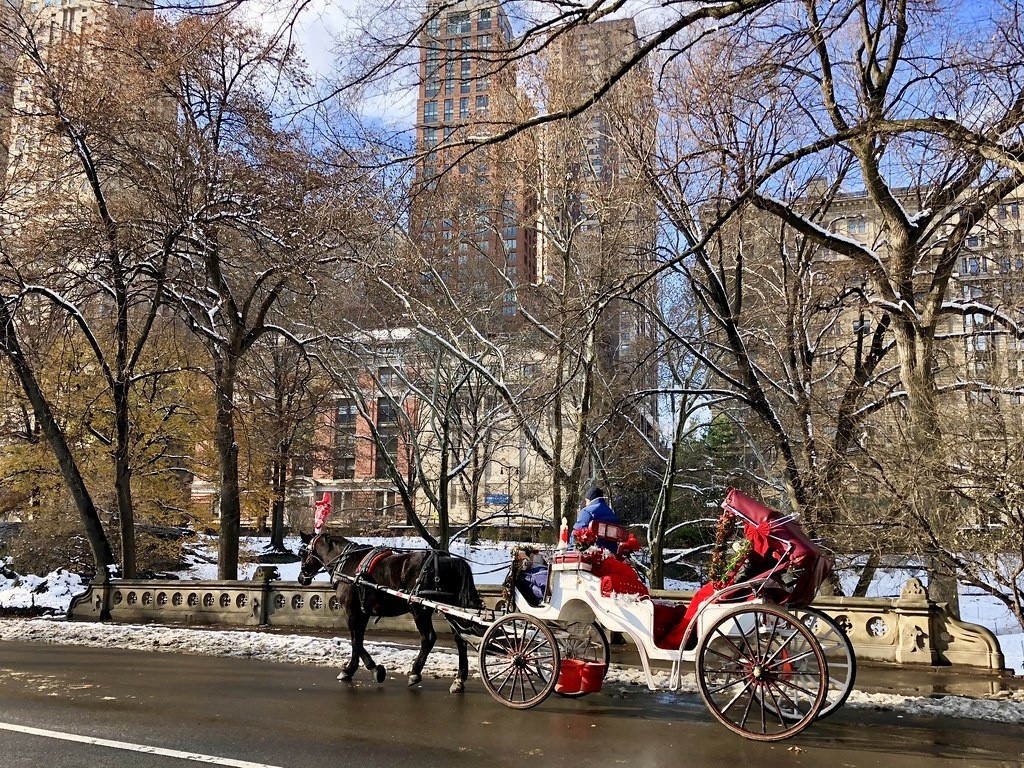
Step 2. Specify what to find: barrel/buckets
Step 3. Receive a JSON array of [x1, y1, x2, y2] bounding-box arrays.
[[552, 658, 584, 694], [580, 659, 607, 692]]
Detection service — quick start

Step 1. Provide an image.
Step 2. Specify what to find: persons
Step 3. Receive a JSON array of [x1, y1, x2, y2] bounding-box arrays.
[[566, 487, 617, 553]]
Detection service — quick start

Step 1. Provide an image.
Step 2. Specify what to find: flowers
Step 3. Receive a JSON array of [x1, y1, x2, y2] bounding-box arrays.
[[710, 507, 753, 589], [573, 528, 619, 566]]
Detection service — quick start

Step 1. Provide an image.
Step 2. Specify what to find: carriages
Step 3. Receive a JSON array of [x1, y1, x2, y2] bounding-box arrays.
[[298, 487, 859, 745]]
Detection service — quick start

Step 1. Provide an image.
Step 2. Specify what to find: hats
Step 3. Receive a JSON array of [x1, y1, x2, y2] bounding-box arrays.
[[585, 488, 603, 501]]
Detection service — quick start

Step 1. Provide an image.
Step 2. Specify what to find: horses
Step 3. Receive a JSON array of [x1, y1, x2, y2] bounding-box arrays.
[[298, 530, 484, 694]]
